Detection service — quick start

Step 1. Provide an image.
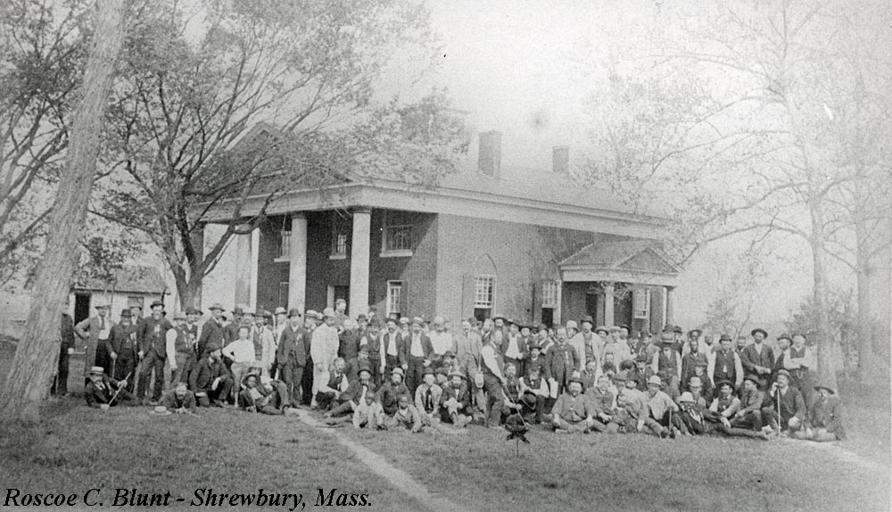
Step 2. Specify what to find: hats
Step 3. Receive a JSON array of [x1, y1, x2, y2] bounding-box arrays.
[[204, 342, 222, 354], [772, 368, 795, 387], [620, 323, 631, 335], [230, 306, 288, 317], [812, 382, 835, 395], [593, 325, 610, 337], [619, 359, 638, 372], [185, 305, 198, 315], [675, 391, 697, 404], [303, 309, 319, 319], [474, 373, 484, 388], [750, 328, 769, 339], [420, 367, 435, 380], [321, 307, 337, 319], [687, 376, 704, 387], [130, 302, 143, 311], [236, 325, 251, 338], [383, 313, 400, 325], [791, 330, 809, 344], [716, 379, 736, 396], [776, 331, 795, 347], [85, 366, 105, 378], [150, 300, 166, 316], [149, 405, 173, 417], [356, 365, 373, 378], [529, 343, 542, 350], [390, 367, 406, 379], [647, 374, 662, 388], [356, 314, 369, 322], [519, 394, 537, 411], [490, 313, 508, 323], [659, 323, 702, 344], [446, 370, 468, 382], [718, 334, 733, 344], [94, 296, 112, 309], [240, 361, 263, 390], [743, 373, 761, 389], [505, 317, 549, 335], [580, 315, 595, 326], [207, 302, 227, 321], [409, 316, 426, 329], [118, 309, 134, 318], [565, 320, 581, 332], [287, 308, 302, 319]]
